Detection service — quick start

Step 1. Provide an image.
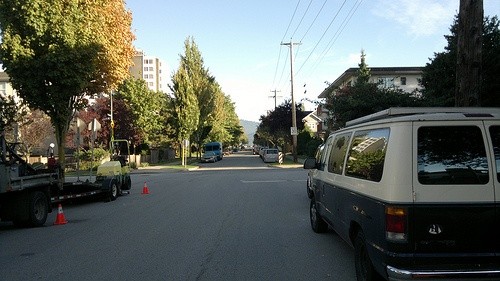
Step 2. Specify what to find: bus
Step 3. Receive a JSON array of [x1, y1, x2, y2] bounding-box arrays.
[[202, 142, 223, 161]]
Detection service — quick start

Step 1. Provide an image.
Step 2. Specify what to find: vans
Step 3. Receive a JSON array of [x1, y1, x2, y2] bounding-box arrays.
[[254, 146, 279, 162], [302, 106, 500, 281]]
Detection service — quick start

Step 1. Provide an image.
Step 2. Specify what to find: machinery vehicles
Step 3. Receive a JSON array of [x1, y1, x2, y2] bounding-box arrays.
[[1, 114, 132, 228]]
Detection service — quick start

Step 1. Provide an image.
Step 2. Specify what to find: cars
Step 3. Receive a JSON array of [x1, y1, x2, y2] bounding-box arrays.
[[201, 155, 218, 163]]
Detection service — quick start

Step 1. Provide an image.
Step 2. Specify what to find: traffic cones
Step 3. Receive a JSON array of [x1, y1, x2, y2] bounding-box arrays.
[[54, 204, 67, 225], [143, 180, 149, 194]]
